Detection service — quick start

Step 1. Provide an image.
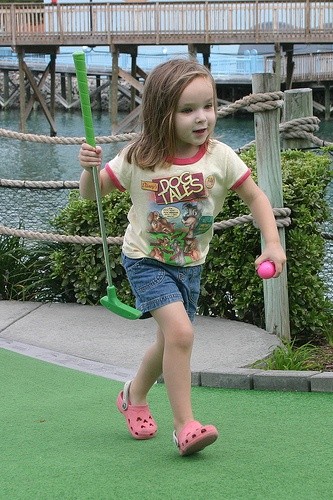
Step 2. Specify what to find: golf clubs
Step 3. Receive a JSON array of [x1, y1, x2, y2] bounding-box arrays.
[[72, 50, 144, 321]]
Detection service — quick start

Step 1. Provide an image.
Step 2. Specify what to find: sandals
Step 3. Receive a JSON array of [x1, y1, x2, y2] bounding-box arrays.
[[116, 379, 158, 440], [172, 418, 219, 457]]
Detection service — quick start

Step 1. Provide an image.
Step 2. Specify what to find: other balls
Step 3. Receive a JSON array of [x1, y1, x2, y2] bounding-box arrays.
[[258, 261, 275, 279]]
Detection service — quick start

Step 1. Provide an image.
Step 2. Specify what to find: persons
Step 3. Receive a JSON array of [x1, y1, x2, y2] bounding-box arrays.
[[78, 59, 286, 453]]
